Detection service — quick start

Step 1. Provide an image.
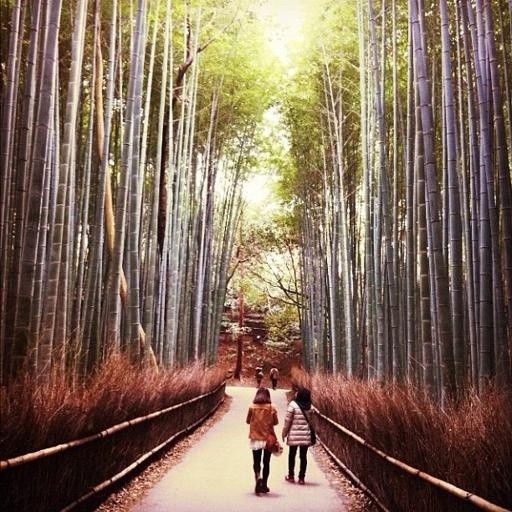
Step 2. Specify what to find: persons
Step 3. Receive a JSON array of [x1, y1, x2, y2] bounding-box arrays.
[[282, 388, 312, 485], [246, 387, 278, 493], [254, 364, 262, 388], [269, 364, 279, 390]]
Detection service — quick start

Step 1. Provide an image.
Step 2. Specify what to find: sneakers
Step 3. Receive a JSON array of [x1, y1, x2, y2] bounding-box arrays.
[[285, 475, 304, 484], [256, 479, 269, 493]]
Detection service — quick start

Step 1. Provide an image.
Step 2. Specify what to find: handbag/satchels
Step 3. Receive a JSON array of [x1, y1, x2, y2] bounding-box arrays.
[[309, 426, 316, 444], [266, 434, 276, 452]]
